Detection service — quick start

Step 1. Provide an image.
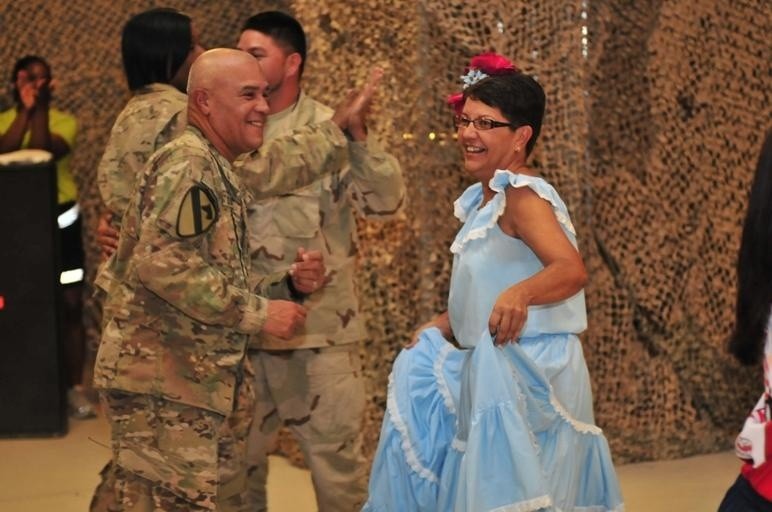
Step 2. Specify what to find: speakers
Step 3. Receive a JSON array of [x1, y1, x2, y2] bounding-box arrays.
[[0, 163, 68, 441]]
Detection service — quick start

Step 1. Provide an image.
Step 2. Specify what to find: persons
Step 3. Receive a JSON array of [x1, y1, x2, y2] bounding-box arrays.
[[717, 127, 772, 511], [87, 46, 329, 512], [236, 9, 404, 512], [1, 54, 94, 421], [94, 7, 213, 325], [359, 54, 628, 512]]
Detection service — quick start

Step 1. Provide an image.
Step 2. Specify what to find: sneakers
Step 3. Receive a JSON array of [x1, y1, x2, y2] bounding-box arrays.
[[67, 386, 98, 419]]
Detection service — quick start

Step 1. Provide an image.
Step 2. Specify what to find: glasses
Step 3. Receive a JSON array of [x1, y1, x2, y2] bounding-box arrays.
[[454, 111, 512, 131]]
